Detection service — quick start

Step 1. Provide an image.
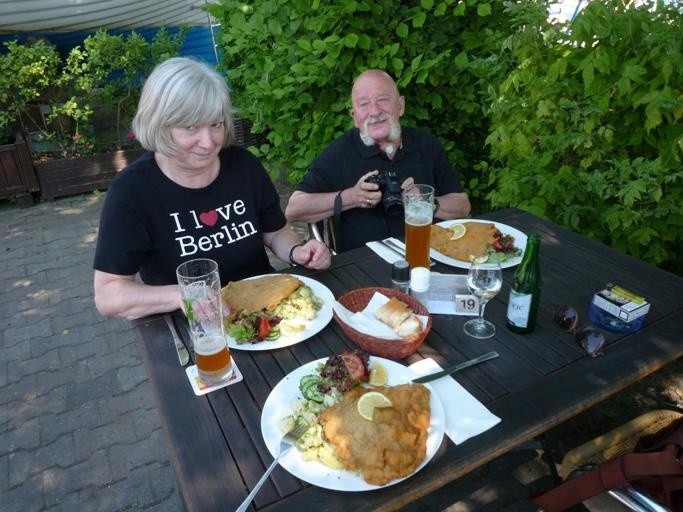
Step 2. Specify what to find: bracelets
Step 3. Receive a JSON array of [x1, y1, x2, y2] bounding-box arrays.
[[428, 197, 441, 215], [286, 240, 305, 270]]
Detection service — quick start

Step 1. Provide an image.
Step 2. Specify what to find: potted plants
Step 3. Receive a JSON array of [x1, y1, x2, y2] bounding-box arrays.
[[0, 17, 192, 204]]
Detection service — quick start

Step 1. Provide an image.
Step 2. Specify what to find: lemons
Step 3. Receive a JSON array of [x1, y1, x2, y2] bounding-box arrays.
[[449, 223, 466, 240], [469, 255, 488, 263], [368, 362, 387, 386], [357, 392, 392, 420]]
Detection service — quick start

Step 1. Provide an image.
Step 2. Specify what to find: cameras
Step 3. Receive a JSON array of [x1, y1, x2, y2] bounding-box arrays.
[[366, 170, 404, 216]]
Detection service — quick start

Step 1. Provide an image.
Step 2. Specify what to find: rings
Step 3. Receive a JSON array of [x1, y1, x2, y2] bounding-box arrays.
[[367, 199, 374, 210]]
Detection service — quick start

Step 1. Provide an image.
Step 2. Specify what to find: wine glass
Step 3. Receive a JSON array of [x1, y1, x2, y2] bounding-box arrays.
[[460, 255, 504, 338]]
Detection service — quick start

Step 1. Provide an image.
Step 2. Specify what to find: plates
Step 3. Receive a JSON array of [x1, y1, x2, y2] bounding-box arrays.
[[222, 273, 334, 352], [429, 219, 531, 271], [259, 354, 447, 494]]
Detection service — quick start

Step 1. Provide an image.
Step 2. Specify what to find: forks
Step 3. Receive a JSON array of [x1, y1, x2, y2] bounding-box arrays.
[[233, 419, 308, 512]]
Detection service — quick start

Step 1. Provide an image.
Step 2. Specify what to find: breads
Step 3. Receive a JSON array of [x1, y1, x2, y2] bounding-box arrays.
[[376, 296, 422, 339]]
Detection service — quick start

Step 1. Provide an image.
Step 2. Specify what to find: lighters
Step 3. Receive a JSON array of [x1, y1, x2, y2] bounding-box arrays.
[[605, 280, 646, 305]]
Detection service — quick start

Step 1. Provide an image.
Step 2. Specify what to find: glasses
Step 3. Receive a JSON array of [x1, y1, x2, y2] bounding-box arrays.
[[550, 304, 610, 360]]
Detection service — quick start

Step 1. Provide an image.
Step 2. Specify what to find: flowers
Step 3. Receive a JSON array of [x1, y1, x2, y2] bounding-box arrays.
[[125, 131, 137, 148], [72, 134, 96, 156]]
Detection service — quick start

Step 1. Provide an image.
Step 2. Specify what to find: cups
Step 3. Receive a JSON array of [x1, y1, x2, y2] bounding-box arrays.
[[174, 259, 233, 385], [402, 183, 434, 270]]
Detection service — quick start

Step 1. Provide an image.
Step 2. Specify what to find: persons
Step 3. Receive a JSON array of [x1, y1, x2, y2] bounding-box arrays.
[[283, 67, 473, 254], [91, 55, 331, 331]]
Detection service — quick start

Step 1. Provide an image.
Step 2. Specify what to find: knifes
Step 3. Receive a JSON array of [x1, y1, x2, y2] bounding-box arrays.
[[409, 348, 504, 386], [162, 315, 190, 368]]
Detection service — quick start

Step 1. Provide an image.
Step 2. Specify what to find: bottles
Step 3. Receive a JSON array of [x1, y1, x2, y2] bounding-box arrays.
[[392, 259, 431, 311], [505, 232, 545, 334]]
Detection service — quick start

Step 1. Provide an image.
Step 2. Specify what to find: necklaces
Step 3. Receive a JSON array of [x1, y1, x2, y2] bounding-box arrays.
[[379, 142, 396, 158]]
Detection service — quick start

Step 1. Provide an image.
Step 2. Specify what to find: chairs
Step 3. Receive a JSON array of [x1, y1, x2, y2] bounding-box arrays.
[[307, 211, 337, 255], [535, 408, 681, 510]]
